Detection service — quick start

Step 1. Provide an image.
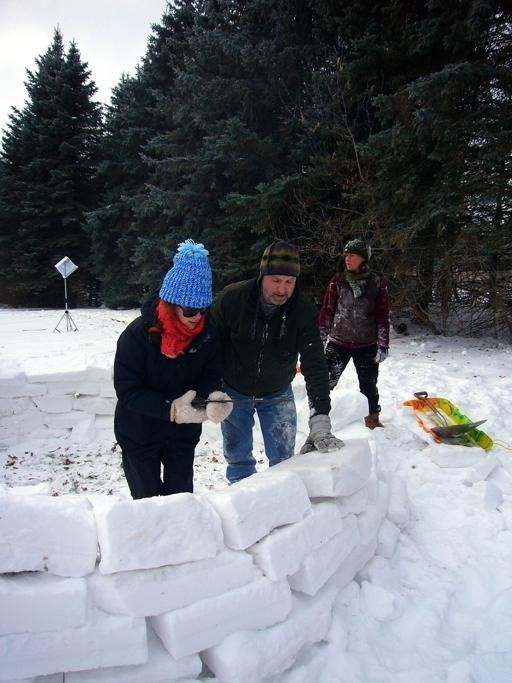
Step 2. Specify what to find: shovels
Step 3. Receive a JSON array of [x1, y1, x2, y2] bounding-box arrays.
[[414, 392, 488, 439]]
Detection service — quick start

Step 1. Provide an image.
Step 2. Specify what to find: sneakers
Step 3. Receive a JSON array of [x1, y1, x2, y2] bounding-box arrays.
[[365, 415, 384, 430]]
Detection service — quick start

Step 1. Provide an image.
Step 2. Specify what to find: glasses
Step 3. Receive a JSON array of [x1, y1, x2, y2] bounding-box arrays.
[[177, 305, 207, 317]]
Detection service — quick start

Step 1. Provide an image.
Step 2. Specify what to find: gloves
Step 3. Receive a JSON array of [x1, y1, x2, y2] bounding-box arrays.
[[206, 390, 234, 424], [170, 390, 208, 424], [300, 415, 344, 454], [375, 345, 389, 363]]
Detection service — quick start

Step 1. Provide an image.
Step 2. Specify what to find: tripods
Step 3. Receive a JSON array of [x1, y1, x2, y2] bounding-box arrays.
[[52, 262, 79, 333]]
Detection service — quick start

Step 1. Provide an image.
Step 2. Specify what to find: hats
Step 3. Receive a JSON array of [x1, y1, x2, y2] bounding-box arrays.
[[343, 238, 372, 262], [159, 239, 212, 309], [257, 239, 300, 284]]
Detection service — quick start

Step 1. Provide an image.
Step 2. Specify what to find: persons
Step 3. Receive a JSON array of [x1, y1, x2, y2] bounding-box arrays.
[[212, 238, 346, 485], [319, 237, 393, 430], [113, 238, 233, 500]]
